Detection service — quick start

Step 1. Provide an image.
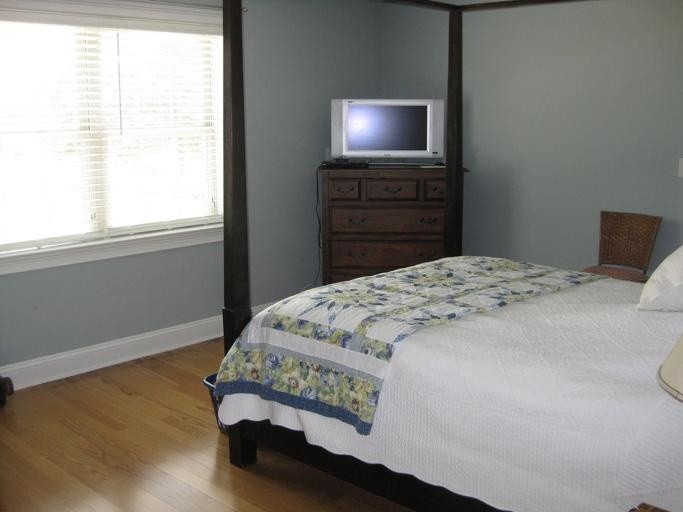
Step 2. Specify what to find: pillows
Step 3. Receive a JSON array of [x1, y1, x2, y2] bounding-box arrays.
[[633, 243, 683, 310], [656, 334, 682, 406]]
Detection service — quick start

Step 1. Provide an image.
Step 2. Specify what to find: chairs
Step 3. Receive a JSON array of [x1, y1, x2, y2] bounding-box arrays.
[[579, 208, 663, 284]]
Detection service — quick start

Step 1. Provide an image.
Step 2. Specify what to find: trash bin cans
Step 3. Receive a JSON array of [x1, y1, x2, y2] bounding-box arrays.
[[204, 373, 229, 433]]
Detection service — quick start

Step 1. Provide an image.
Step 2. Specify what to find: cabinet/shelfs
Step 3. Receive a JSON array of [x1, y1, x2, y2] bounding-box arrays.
[[318, 160, 465, 283]]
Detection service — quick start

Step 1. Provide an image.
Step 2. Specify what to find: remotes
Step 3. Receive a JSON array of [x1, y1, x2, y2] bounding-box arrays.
[[327, 162, 369, 169]]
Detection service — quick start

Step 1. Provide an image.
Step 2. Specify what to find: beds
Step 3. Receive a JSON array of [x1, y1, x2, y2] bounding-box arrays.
[[217, 255, 682, 512]]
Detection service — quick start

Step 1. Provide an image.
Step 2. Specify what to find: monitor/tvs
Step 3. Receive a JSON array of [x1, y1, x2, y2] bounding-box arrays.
[[331, 99, 444, 166]]
